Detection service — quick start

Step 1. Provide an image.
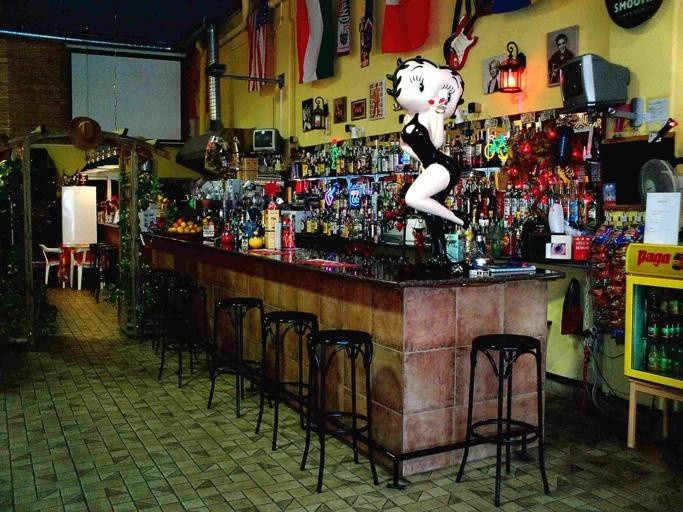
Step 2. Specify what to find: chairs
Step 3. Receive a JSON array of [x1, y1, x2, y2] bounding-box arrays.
[[70, 247, 98, 291], [37, 243, 66, 289]]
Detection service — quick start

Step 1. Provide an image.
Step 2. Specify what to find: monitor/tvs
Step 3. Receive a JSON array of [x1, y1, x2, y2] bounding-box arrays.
[[559, 52, 631, 108], [252, 127, 281, 152]]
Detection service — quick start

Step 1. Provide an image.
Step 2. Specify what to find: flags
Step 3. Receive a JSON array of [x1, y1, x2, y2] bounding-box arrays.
[[382, 0, 431, 54], [247, 0, 269, 92], [296, 0, 334, 84]]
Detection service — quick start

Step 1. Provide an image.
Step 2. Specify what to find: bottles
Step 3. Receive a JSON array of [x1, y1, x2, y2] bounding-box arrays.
[[444, 178, 597, 257], [643, 289, 683, 378], [304, 190, 382, 244], [289, 115, 540, 177]]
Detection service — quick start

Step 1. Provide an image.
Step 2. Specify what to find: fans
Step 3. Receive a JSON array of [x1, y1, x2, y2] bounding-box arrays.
[[638, 158, 683, 208]]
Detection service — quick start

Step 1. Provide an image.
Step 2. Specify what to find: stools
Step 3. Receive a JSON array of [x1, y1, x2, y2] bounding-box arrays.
[[625, 376, 683, 449], [455, 333, 551, 507], [90, 243, 211, 392], [207, 297, 270, 420], [300, 330, 380, 493], [254, 311, 319, 450]]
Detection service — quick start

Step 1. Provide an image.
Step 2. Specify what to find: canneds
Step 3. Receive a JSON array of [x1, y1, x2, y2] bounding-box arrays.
[[281, 250, 295, 264], [290, 160, 302, 179], [265, 209, 296, 250]]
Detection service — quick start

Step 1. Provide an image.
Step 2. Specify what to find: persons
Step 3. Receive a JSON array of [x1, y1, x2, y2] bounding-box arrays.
[[372, 83, 380, 117], [487, 59, 500, 93], [302, 104, 312, 132], [548, 33, 574, 83], [385, 55, 470, 263]]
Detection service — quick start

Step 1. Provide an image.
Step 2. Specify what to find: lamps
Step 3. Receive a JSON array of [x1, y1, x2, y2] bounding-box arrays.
[[310, 96, 329, 130], [498, 42, 527, 94]]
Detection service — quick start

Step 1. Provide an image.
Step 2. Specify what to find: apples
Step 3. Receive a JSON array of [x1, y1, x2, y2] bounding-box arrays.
[[167, 218, 202, 234]]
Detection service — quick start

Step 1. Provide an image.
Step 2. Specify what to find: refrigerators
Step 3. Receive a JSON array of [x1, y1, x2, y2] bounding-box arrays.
[[624, 244, 683, 389]]
